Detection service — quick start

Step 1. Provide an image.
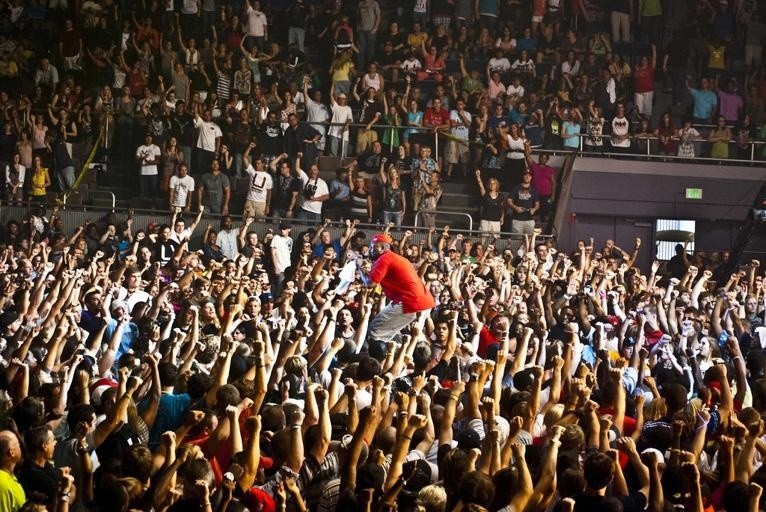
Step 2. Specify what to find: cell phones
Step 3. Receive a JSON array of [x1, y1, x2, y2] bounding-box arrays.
[[718, 331, 729, 348]]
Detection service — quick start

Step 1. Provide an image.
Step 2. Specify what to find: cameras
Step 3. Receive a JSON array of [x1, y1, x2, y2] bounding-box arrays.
[[523, 209, 530, 211]]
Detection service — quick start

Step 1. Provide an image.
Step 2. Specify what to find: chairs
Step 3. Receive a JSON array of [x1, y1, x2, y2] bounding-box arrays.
[[652, 81, 689, 130], [232, 156, 480, 226], [47, 124, 167, 215]]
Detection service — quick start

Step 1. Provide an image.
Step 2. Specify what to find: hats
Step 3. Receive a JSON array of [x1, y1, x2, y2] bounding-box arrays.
[[370, 234, 394, 245], [279, 222, 292, 230]]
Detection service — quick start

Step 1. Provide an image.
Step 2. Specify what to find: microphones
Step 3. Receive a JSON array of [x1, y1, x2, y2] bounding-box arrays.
[[351, 253, 369, 262]]
